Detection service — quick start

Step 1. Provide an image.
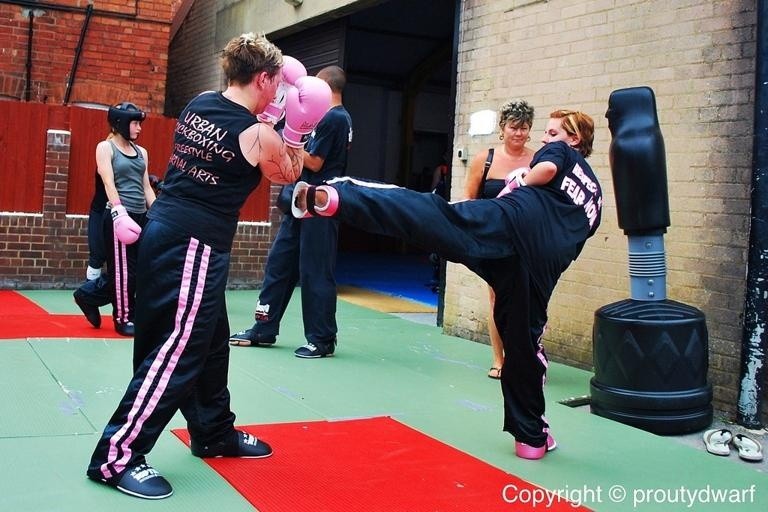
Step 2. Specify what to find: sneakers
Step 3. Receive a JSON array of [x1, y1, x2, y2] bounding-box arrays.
[[72, 289, 102, 329], [192, 427, 273, 461], [114, 319, 136, 336], [295, 340, 335, 359], [90, 457, 173, 499], [231, 329, 276, 348]]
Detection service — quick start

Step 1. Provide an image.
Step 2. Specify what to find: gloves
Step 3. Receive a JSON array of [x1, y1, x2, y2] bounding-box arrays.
[[505, 167, 531, 190], [283, 76, 332, 148], [258, 55, 307, 123], [110, 203, 142, 244]]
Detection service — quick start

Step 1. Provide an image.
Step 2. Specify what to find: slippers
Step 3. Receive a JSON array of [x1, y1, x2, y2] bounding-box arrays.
[[732, 434, 765, 462], [488, 367, 502, 379], [701, 426, 734, 458]]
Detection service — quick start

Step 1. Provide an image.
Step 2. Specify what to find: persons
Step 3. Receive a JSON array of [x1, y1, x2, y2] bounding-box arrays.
[[226, 64, 354, 358], [84, 31, 332, 500], [74, 99, 157, 335], [85, 123, 116, 282], [284, 109, 601, 459], [462, 101, 544, 379], [424, 152, 448, 293]]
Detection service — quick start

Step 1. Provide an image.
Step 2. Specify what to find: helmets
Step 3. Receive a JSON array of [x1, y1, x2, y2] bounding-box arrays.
[[107, 102, 146, 141]]
